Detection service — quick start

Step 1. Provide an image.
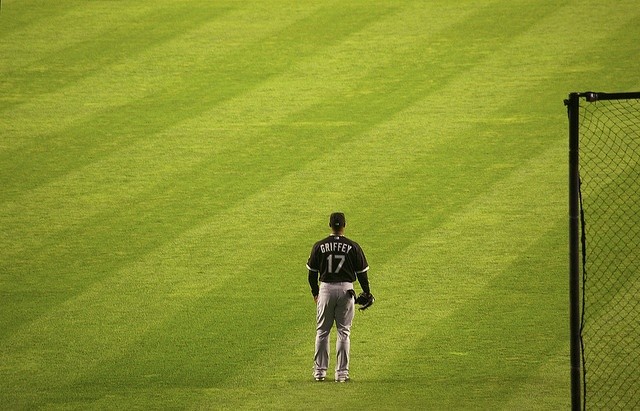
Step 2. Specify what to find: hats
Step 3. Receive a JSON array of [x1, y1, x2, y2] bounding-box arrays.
[[330, 212, 345, 226]]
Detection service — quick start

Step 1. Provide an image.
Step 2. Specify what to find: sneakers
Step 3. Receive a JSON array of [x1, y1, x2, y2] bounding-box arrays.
[[335, 375, 348, 383], [314, 374, 325, 380]]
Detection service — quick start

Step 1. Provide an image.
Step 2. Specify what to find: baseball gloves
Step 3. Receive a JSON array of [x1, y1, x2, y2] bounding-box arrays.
[[356, 292, 375, 310]]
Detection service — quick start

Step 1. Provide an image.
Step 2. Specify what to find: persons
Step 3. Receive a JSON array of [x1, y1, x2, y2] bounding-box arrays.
[[305, 210, 377, 380]]
